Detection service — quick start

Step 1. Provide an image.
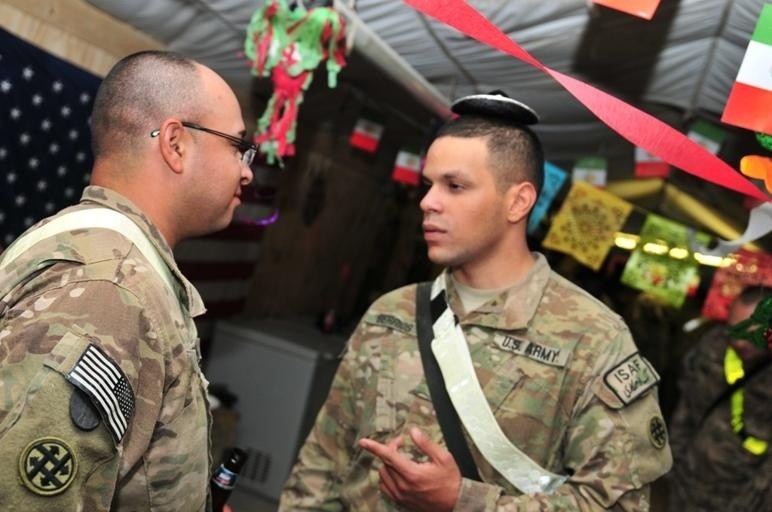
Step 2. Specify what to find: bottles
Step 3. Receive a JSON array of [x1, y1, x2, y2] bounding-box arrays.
[[316, 264, 351, 336], [210, 446, 249, 512]]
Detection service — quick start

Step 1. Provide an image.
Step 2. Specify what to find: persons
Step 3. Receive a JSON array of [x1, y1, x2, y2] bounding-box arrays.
[[276, 87, 676, 512], [0, 49, 254, 511], [658, 281, 772, 512]]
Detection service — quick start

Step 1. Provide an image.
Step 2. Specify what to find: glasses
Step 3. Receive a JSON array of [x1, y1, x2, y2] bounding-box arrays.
[[151, 120, 258, 167]]
